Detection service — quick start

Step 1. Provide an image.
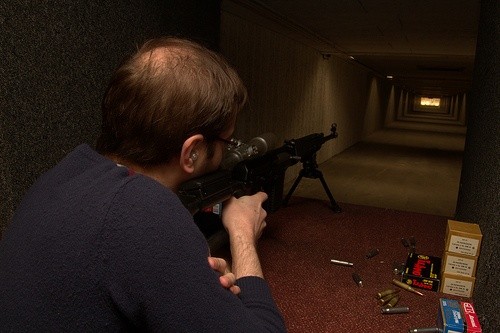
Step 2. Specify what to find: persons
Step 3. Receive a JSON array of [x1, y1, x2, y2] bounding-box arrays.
[[0, 35, 288, 333]]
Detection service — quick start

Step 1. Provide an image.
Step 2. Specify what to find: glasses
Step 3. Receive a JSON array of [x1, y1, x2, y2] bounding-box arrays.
[[212, 133, 238, 150]]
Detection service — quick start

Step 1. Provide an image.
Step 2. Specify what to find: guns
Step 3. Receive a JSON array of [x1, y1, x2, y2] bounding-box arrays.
[[175, 121, 343, 215]]
